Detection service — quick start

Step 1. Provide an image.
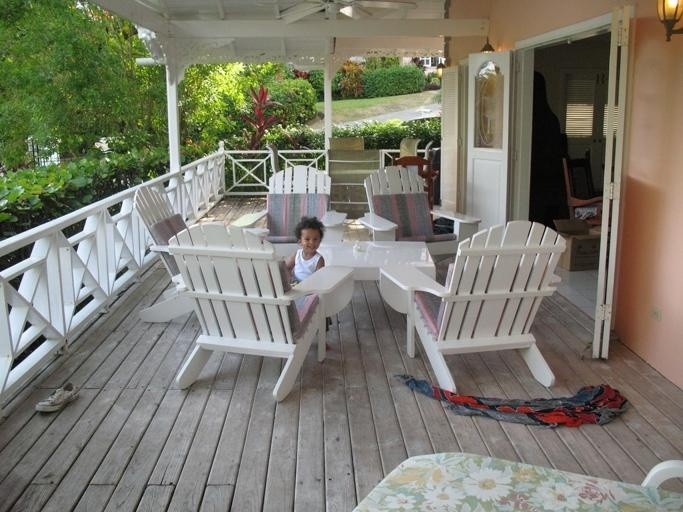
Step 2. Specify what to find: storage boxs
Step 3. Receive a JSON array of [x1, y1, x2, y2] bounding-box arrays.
[[552, 218, 602, 271]]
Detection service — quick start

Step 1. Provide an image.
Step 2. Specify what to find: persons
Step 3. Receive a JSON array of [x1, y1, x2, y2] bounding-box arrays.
[[284, 217, 334, 351]]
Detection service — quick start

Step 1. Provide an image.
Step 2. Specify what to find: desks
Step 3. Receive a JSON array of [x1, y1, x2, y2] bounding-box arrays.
[[259, 242, 437, 358], [429, 210, 482, 242]]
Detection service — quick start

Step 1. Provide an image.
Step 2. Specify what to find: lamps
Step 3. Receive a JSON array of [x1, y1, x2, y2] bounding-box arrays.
[[436, 58, 444, 79], [653, 0, 683, 43], [480, 36, 494, 54]]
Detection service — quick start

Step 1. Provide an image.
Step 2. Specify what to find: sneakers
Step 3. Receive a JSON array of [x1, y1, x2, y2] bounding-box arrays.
[[35, 382, 79, 413]]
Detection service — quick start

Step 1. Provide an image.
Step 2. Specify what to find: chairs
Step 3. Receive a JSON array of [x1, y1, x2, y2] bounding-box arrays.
[[561, 156, 611, 235], [351, 452, 682, 511], [230, 165, 347, 245], [378, 219, 567, 394], [392, 153, 438, 220], [132, 178, 269, 322], [167, 222, 353, 402], [358, 165, 482, 256]]
